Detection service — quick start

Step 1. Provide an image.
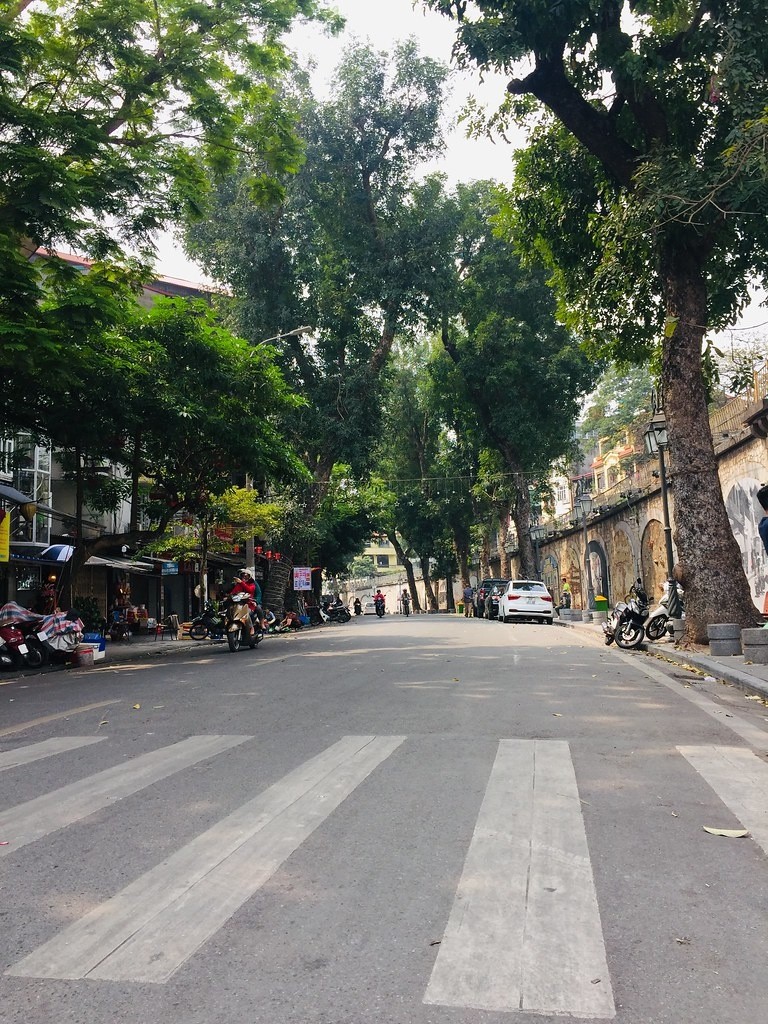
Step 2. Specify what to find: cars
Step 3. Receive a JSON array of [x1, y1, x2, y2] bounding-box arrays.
[[485, 584, 507, 620], [364, 602, 376, 615], [498, 581, 553, 625]]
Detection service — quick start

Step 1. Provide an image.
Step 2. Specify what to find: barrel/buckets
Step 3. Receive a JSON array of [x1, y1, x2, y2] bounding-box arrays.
[[76, 645, 94, 666]]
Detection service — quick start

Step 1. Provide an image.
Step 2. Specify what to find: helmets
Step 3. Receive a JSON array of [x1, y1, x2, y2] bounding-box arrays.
[[238, 568, 252, 580], [377, 590, 381, 593], [403, 589, 407, 592]]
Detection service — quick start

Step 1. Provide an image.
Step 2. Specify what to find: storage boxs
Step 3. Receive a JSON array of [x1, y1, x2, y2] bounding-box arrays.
[[126, 603, 158, 629]]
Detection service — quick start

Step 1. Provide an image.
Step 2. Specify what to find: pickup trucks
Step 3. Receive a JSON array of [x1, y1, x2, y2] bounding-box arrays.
[[473, 577, 509, 618]]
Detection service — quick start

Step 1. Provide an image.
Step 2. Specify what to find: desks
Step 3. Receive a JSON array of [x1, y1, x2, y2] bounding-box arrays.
[[114, 622, 133, 644]]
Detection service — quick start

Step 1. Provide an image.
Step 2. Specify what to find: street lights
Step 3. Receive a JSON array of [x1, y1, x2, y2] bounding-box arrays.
[[246, 326, 312, 581], [573, 493, 597, 621], [643, 382, 683, 639], [469, 555, 481, 590], [529, 523, 543, 581]]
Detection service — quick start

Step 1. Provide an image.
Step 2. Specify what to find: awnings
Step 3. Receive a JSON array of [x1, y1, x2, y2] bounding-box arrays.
[[85, 554, 179, 576]]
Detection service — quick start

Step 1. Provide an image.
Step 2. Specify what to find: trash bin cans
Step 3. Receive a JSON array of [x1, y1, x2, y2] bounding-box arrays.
[[75, 645, 94, 668], [594, 596, 608, 611], [457, 603, 464, 613]]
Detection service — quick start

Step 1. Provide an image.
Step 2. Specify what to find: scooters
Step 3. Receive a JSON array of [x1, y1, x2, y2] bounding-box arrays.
[[371, 594, 386, 618], [219, 590, 264, 652], [398, 597, 412, 617], [355, 602, 360, 614], [646, 579, 685, 641]]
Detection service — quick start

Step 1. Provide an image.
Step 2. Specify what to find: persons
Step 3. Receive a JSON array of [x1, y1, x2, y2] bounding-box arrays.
[[464, 584, 474, 618], [547, 578, 572, 618], [225, 569, 413, 635], [757, 485, 768, 560]]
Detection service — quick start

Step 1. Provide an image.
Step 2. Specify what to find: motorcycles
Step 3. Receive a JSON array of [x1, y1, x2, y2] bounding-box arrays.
[[188, 594, 227, 641], [601, 577, 654, 649], [0, 600, 85, 674], [310, 594, 351, 626]]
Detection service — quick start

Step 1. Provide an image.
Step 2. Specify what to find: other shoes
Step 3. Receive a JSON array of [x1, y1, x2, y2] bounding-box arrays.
[[250, 629, 255, 635], [261, 625, 266, 630]]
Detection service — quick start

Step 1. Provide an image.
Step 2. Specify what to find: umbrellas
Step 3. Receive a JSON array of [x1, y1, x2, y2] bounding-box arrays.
[[40, 544, 80, 590]]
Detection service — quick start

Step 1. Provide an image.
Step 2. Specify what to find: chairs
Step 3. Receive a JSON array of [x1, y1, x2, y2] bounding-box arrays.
[[155, 615, 180, 642]]
